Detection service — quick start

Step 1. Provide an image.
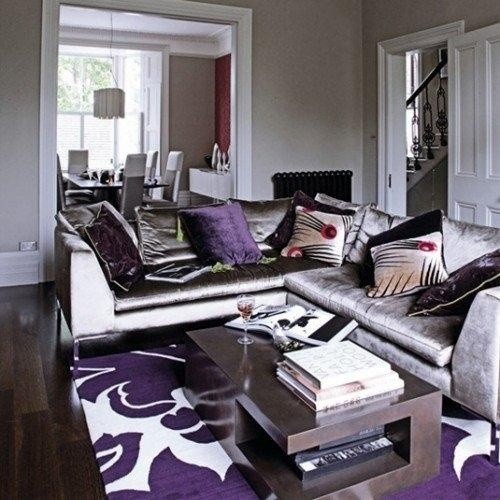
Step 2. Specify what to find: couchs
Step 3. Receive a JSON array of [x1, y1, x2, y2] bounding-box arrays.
[[46, 216, 500, 468]]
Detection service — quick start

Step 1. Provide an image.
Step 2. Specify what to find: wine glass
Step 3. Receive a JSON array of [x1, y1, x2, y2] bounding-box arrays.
[[237, 293, 255, 345]]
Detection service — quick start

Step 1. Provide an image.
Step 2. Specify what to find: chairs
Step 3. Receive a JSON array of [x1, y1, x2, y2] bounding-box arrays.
[[56, 149, 185, 218]]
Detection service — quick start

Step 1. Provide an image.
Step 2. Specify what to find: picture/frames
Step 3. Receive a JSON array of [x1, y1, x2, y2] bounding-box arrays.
[[438, 47, 449, 78]]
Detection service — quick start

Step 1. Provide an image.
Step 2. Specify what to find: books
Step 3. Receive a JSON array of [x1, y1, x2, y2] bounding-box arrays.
[[145, 262, 212, 283], [225, 304, 405, 413]]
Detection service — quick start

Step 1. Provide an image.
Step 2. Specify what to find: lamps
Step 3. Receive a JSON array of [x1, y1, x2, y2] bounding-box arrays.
[[95, 11, 126, 120]]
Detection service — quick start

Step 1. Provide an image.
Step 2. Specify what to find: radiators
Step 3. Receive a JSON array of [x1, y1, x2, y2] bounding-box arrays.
[[271, 169, 354, 203]]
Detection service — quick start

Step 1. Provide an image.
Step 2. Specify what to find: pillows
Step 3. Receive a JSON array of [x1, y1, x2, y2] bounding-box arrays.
[[259, 190, 371, 268], [355, 209, 500, 315], [55, 198, 264, 292]]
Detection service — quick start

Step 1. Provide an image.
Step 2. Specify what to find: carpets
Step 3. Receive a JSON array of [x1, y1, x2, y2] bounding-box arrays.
[[71, 342, 498, 500]]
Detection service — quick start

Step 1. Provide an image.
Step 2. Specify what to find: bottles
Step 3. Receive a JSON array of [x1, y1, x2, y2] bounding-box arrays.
[[108, 158, 116, 186]]
